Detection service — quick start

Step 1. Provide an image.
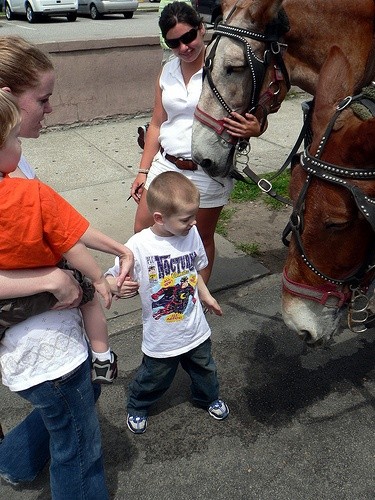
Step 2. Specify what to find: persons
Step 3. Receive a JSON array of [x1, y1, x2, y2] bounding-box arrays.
[[130, 2, 268, 314], [105, 172, 230, 435], [0, 36, 135, 500]]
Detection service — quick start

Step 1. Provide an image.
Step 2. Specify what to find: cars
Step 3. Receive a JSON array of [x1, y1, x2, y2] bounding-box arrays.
[[77, 1, 140, 20], [4, 0, 80, 23]]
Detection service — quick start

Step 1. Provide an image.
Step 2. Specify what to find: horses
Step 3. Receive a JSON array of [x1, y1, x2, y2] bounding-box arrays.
[[278, 44, 375, 350], [192, 0, 375, 213]]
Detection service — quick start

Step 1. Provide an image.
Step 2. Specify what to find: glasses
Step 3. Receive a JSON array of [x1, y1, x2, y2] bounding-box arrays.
[[164, 26, 202, 49]]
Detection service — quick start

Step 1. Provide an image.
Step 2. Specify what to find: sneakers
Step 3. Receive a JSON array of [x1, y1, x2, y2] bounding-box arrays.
[[90, 350, 118, 384], [126, 412, 148, 435], [207, 399, 230, 420]]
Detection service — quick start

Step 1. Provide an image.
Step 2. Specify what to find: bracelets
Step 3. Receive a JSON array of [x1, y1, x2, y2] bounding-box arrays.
[[138, 168, 149, 174]]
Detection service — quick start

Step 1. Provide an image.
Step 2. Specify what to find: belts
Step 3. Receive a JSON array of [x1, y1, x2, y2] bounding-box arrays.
[[158, 143, 199, 173]]
